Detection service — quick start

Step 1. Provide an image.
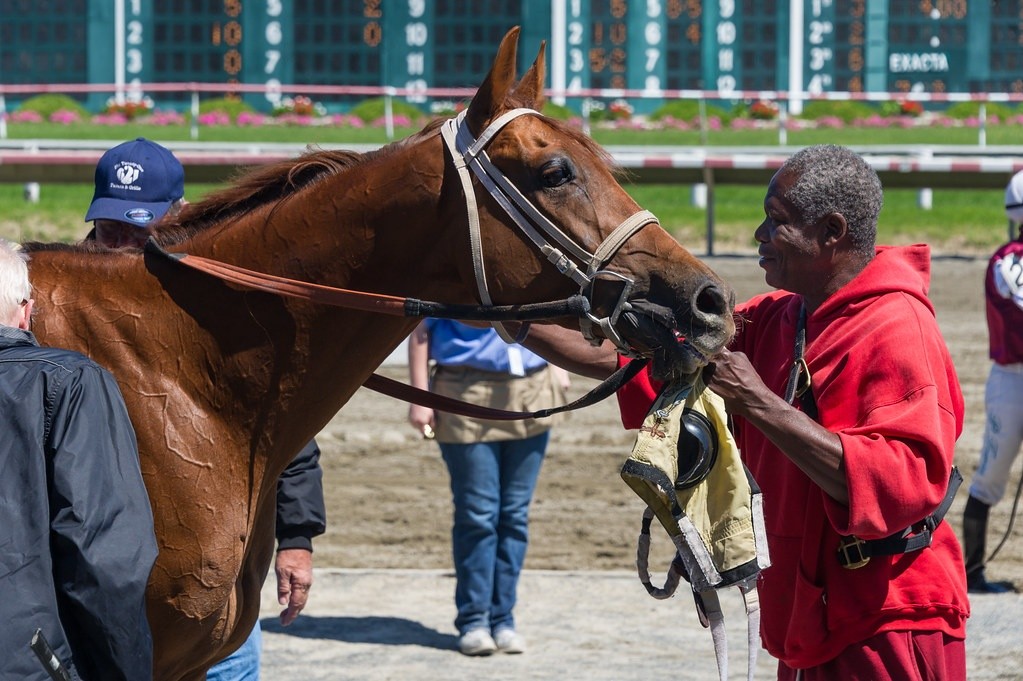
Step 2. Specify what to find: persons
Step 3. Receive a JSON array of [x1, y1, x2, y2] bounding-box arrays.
[[0, 239, 159, 681], [81, 138, 327, 681], [408, 317, 571, 655], [963, 169, 1023, 593], [456, 145, 970, 681]]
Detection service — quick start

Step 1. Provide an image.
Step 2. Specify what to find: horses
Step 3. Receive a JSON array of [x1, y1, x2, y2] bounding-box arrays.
[[22, 25, 735, 681]]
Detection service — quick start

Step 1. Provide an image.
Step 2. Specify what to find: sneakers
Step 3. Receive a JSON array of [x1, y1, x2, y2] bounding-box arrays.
[[492, 629, 524, 652], [457, 629, 497, 655]]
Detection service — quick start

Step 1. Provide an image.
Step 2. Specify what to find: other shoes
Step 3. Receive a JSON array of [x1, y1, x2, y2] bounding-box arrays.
[[966, 566, 986, 591]]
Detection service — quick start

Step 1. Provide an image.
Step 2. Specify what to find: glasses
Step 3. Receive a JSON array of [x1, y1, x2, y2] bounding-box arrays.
[[95, 220, 146, 245]]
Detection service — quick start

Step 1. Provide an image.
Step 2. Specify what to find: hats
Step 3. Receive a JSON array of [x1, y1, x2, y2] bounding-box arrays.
[[1004, 169, 1023, 223], [85, 137, 184, 227]]
